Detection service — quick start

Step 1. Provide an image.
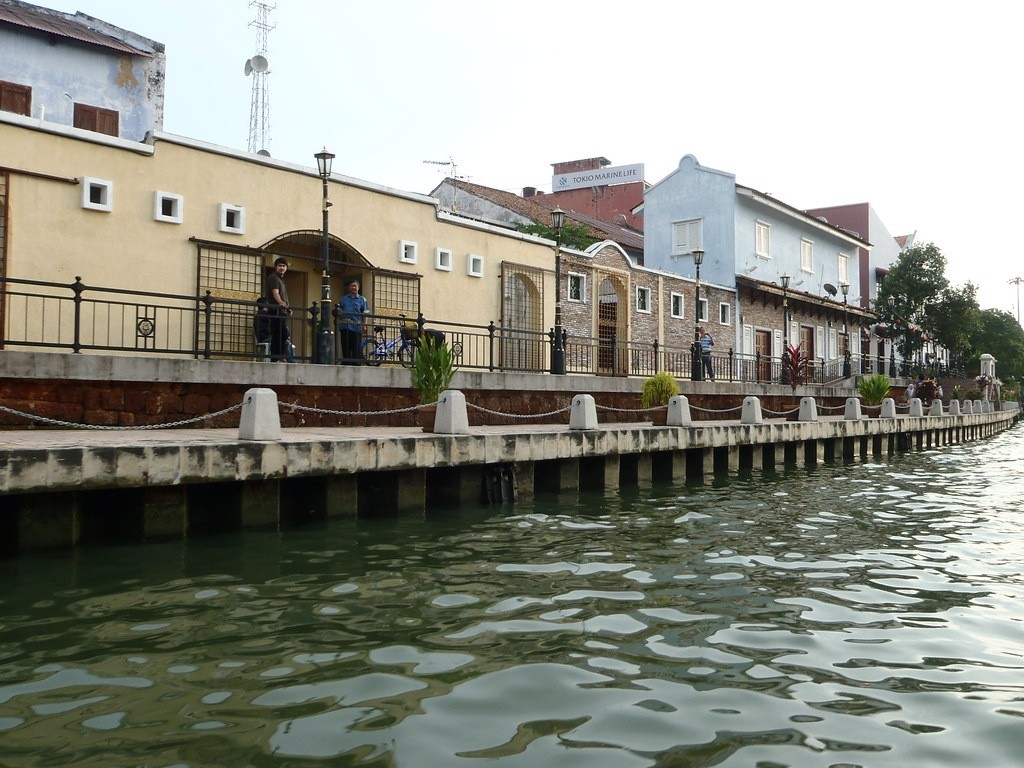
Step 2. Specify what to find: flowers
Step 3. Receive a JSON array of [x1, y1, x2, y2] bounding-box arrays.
[[951, 385, 961, 399]]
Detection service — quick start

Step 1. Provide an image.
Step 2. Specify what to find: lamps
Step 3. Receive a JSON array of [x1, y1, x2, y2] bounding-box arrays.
[[792, 279, 805, 286], [745, 264, 757, 273], [852, 296, 862, 302]]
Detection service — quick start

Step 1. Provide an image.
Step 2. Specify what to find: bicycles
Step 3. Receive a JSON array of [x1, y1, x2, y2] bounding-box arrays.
[[360, 314, 421, 368]]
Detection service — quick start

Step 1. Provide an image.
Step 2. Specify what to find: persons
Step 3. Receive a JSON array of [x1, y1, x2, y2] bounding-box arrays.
[[699, 327, 715, 381], [936, 357, 942, 376], [338, 279, 370, 365], [934, 384, 943, 399], [417, 326, 445, 368], [906, 374, 926, 404], [254, 258, 296, 363]]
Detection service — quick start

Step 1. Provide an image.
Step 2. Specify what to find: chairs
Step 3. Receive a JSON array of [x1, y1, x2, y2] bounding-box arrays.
[[251, 327, 292, 363]]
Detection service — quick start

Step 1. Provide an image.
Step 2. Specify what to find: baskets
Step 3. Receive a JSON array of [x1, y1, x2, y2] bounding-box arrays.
[[403, 325, 418, 339]]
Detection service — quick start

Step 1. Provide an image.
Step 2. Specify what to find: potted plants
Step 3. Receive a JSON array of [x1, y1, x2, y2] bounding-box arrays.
[[639, 370, 679, 426], [989, 378, 1000, 411], [779, 340, 813, 421], [914, 381, 939, 415], [856, 374, 892, 418], [999, 376, 1021, 410], [404, 333, 459, 433]]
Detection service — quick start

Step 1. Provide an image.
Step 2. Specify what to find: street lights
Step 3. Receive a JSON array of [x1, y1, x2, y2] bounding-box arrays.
[[693, 247, 703, 382], [841, 283, 851, 378], [550, 204, 564, 349], [780, 275, 789, 385], [315, 144, 334, 350], [888, 295, 896, 378]]
